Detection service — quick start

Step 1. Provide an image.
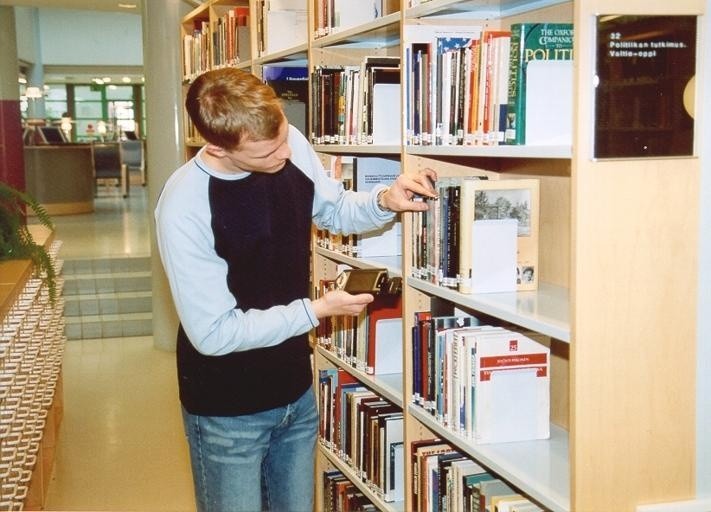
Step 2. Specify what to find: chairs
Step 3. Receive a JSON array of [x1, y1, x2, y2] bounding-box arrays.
[[22, 125, 145, 198]]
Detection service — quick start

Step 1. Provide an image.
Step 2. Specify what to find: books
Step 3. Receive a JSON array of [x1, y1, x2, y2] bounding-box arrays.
[[411, 176, 540, 293], [313, 152, 402, 256], [321, 469, 381, 512], [261, 59, 309, 143], [262, 2, 385, 55], [405, 22, 572, 146], [317, 367, 404, 502], [182, 7, 250, 140], [411, 297, 552, 444], [409, 439, 546, 512], [309, 56, 403, 147], [313, 264, 403, 375]]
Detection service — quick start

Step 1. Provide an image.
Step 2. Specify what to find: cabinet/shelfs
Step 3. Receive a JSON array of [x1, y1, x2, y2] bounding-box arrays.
[[178, 0, 701, 512]]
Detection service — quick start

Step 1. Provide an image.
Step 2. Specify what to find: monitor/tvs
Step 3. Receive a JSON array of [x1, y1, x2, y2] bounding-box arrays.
[[37, 126, 67, 144], [124, 131, 137, 140]]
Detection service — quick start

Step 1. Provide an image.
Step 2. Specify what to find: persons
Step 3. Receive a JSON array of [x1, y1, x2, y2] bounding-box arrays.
[[153, 68, 437, 512]]
